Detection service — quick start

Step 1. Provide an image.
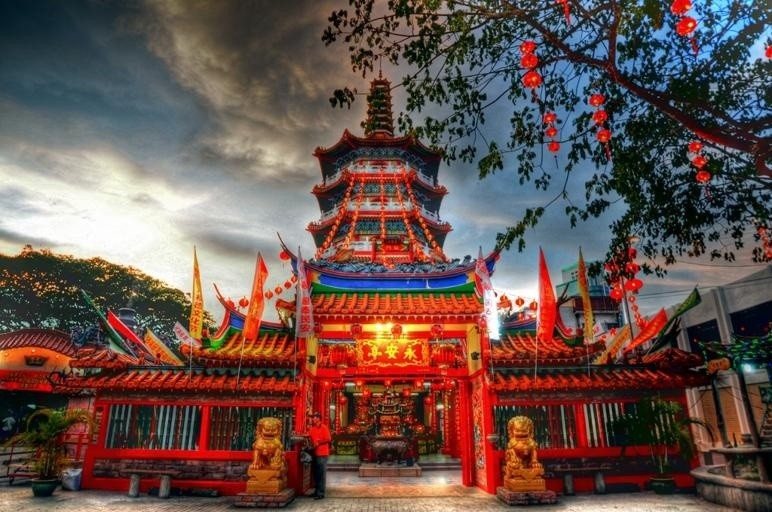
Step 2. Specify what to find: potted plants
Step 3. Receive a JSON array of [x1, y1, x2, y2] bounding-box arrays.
[[607, 396, 714, 494], [2, 408, 97, 497]]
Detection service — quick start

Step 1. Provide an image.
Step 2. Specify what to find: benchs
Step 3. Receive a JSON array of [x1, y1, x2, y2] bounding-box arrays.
[[120, 468, 182, 499], [554, 467, 615, 497]]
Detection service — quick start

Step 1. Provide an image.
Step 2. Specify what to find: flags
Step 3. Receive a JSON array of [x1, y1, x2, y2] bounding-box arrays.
[[172, 321, 197, 349], [142, 325, 187, 369], [535, 247, 557, 342], [298, 246, 314, 342], [476, 250, 502, 341], [188, 248, 204, 339], [237, 250, 269, 340], [107, 307, 163, 367], [593, 323, 633, 364], [622, 305, 669, 355], [80, 289, 134, 363], [639, 287, 703, 355], [576, 249, 594, 340]]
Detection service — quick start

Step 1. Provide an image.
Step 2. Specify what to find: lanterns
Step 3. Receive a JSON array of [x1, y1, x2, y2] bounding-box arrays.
[[222, 271, 297, 311], [601, 248, 646, 325], [543, 112, 560, 155], [521, 41, 543, 99], [683, 136, 710, 184], [668, 0, 692, 17], [676, 16, 700, 52], [588, 91, 612, 161], [313, 168, 447, 267]]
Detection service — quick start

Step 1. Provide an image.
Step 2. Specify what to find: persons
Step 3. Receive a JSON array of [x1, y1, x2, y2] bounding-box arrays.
[[307, 409, 332, 500]]
